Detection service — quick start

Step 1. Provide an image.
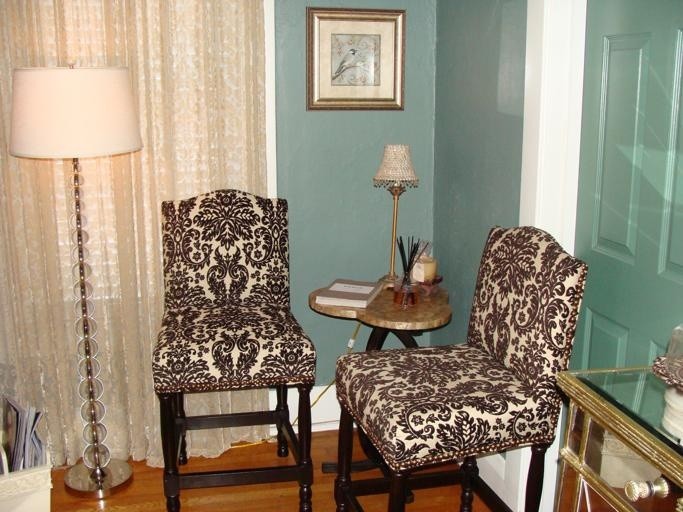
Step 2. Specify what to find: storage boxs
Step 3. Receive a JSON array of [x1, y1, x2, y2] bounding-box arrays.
[[0, 441, 52, 511]]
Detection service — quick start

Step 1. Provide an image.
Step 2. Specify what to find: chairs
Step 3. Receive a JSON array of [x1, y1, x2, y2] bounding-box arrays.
[[334, 225, 587, 512], [151, 189, 316, 512]]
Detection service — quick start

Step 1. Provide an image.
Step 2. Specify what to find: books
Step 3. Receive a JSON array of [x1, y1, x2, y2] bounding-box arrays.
[[315, 278, 383, 309], [0, 395, 46, 475]]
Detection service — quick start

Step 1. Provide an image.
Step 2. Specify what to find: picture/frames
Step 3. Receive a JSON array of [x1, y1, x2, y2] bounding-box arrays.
[[305, 8, 405, 111]]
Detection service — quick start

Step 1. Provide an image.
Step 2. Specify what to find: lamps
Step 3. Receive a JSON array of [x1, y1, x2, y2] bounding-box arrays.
[[7, 64, 143, 499], [371, 143, 419, 291]]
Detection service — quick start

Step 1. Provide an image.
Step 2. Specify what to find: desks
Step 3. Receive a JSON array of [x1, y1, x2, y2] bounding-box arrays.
[[308, 282, 452, 503]]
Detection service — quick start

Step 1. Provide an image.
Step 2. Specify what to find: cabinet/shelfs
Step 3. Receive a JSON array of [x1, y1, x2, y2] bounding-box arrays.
[[552, 364, 683, 512]]
